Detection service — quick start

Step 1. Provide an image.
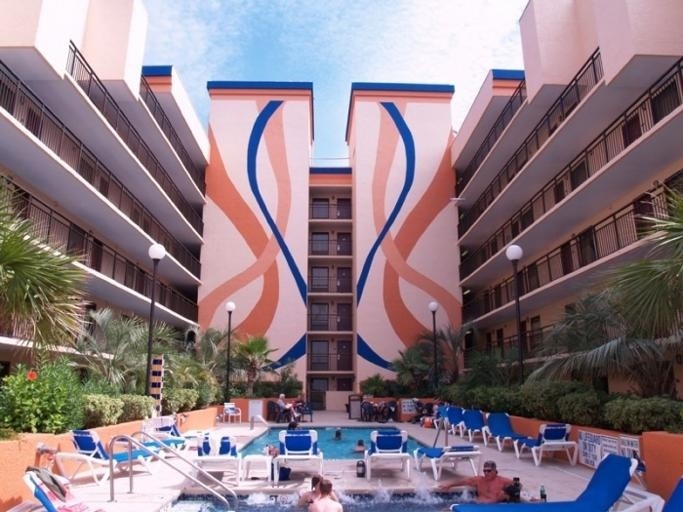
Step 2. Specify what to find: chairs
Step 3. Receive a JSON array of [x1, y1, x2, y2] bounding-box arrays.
[[365, 429, 411, 481], [25, 468, 139, 511], [273, 429, 323, 485], [53, 424, 198, 485], [223, 402, 242, 425], [196, 433, 242, 489], [413, 445, 482, 481], [434, 406, 578, 467], [449, 453, 665, 512], [623, 482, 680, 512]]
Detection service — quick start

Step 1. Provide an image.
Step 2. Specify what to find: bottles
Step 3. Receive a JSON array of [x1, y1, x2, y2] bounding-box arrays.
[[356, 460, 365, 478], [538, 485, 547, 501], [512, 476, 520, 503]]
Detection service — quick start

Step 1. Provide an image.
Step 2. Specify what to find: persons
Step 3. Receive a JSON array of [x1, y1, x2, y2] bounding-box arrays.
[[307, 480, 343, 512], [438, 460, 514, 504], [288, 422, 298, 430], [355, 439, 366, 452], [277, 393, 302, 423], [335, 429, 344, 440], [292, 394, 305, 422], [299, 475, 340, 507], [408, 397, 452, 426]]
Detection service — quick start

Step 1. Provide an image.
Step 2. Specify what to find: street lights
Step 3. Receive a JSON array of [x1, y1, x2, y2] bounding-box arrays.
[[506, 243, 525, 386], [224, 302, 237, 400], [144, 243, 168, 395], [428, 301, 442, 393]]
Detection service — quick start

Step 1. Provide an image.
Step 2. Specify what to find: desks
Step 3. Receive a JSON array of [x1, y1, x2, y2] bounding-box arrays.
[[244, 455, 271, 481]]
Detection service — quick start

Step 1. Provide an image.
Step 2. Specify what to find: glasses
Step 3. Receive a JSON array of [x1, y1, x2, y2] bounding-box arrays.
[[483, 470, 492, 472]]
